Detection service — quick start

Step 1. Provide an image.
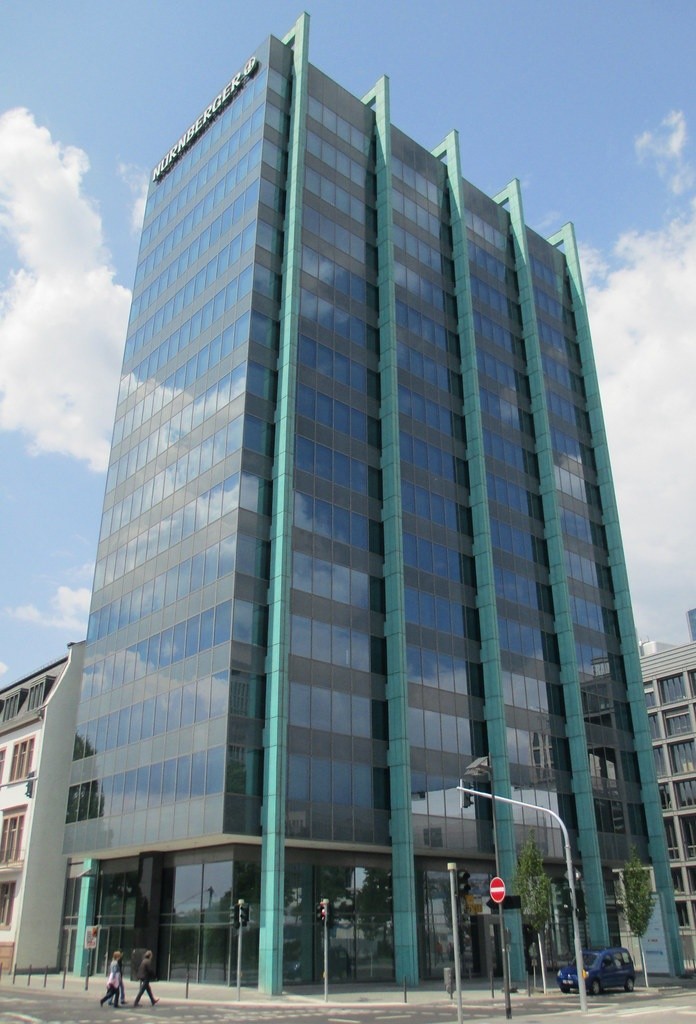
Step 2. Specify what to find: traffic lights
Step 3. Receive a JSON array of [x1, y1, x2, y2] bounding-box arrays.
[[240, 903, 250, 928], [460, 778, 474, 809], [455, 868, 471, 897], [550, 875, 572, 918], [230, 904, 239, 929], [315, 902, 324, 922]]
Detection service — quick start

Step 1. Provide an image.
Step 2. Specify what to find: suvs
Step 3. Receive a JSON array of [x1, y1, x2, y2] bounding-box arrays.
[[556, 946, 637, 996]]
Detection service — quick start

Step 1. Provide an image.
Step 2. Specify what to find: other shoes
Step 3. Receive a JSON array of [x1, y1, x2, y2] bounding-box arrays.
[[109, 1003, 113, 1005], [134, 1005, 142, 1008], [100, 1000, 103, 1006], [114, 1005, 121, 1008], [121, 1001, 127, 1004], [152, 999, 158, 1005]]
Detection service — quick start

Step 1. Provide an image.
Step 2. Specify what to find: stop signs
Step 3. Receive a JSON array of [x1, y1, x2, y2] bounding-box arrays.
[[489, 876, 506, 903]]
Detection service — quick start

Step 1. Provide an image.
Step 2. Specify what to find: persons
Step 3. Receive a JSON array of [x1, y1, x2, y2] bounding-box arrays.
[[434, 940, 455, 963], [98, 949, 129, 1009], [134, 950, 160, 1008]]
